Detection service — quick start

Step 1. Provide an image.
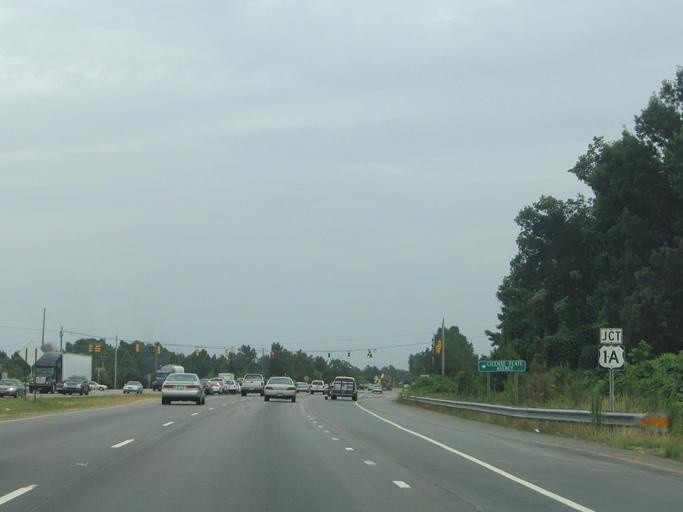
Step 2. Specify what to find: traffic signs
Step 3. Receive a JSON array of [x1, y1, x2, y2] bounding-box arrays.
[[477, 359, 525, 372]]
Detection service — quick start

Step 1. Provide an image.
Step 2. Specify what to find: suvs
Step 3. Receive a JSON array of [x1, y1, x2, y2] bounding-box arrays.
[[60, 374, 90, 396]]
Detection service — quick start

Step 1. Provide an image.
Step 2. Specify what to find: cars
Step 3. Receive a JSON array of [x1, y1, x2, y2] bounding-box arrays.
[[122, 380, 143, 394], [161, 372, 204, 404], [0, 378, 26, 399], [88, 380, 107, 391], [198, 372, 416, 396], [263, 377, 296, 402]]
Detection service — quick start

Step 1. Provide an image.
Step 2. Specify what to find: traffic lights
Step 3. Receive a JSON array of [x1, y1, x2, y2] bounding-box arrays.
[[347, 352, 351, 359], [367, 353, 370, 358]]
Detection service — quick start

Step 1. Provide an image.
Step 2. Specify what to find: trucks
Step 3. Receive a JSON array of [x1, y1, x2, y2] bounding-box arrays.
[[27, 352, 92, 394], [152, 364, 185, 390], [329, 377, 357, 400]]
